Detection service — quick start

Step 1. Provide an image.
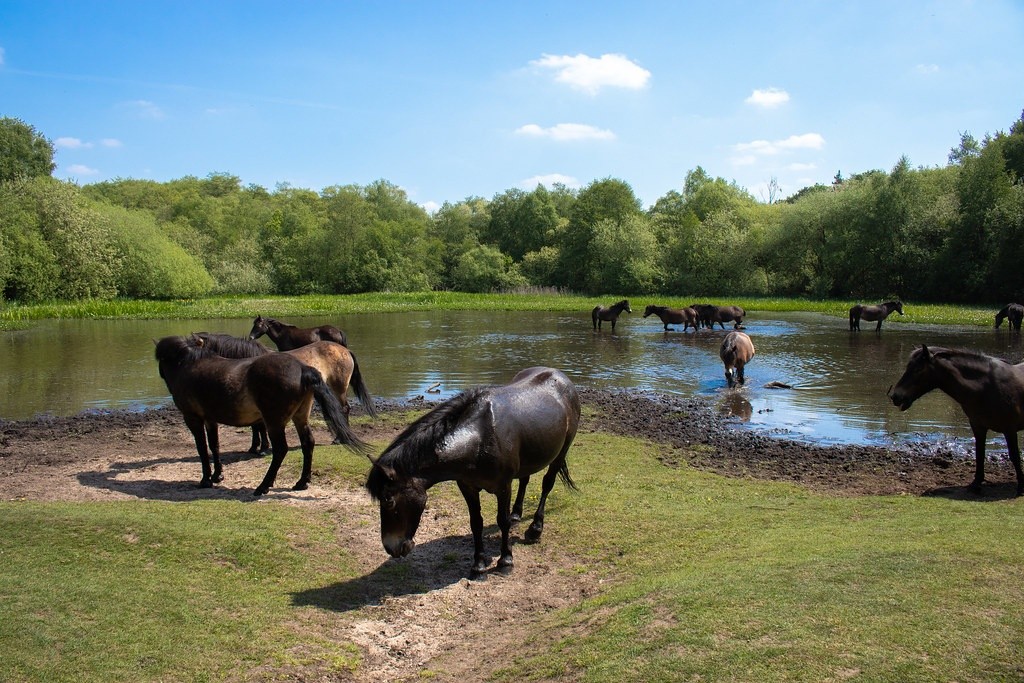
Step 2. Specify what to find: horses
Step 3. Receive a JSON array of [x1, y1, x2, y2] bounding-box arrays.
[[643, 305, 700, 331], [151, 334, 379, 497], [184, 329, 378, 456], [720, 331, 755, 386], [888, 343, 1024, 492], [249, 314, 347, 350], [689, 303, 725, 330], [364, 366, 584, 582], [995, 304, 1024, 331], [849, 301, 904, 332], [720, 305, 747, 330], [592, 300, 632, 332]]
